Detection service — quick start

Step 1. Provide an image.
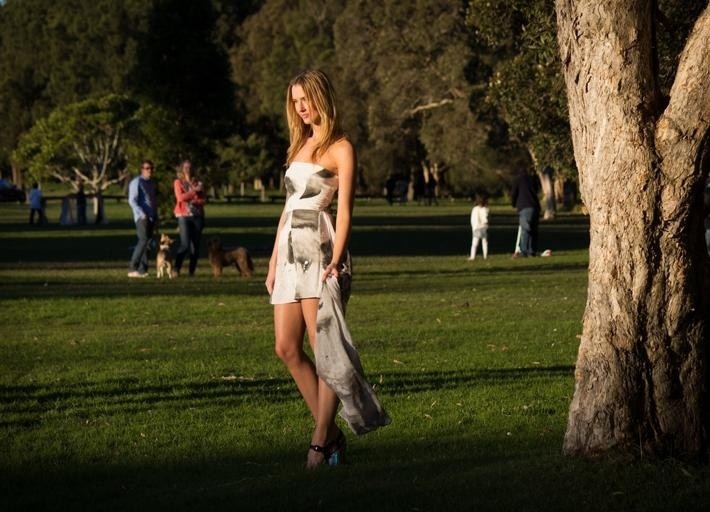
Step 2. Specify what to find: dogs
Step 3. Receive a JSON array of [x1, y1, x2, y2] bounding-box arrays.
[[156, 232, 180, 279], [205, 236, 254, 278]]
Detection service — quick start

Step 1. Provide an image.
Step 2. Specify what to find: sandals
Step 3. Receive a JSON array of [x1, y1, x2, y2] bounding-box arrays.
[[310, 432, 346, 468]]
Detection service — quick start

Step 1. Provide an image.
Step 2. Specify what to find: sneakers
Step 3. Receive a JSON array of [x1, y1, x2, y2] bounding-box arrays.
[[127, 270, 149, 278]]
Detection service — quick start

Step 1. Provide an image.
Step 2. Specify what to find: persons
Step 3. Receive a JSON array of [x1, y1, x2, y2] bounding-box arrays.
[[511, 167, 540, 259], [27, 181, 46, 227], [263, 71, 358, 468], [126, 159, 159, 280], [172, 158, 207, 278], [465, 192, 490, 261]]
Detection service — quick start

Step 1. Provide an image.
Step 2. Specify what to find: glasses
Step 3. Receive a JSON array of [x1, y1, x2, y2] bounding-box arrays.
[[142, 166, 153, 170]]
